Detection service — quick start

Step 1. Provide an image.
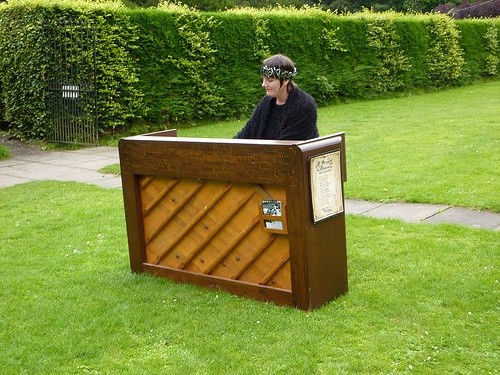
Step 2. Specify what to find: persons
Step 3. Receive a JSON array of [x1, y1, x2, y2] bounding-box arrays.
[[232, 54, 319, 140]]
